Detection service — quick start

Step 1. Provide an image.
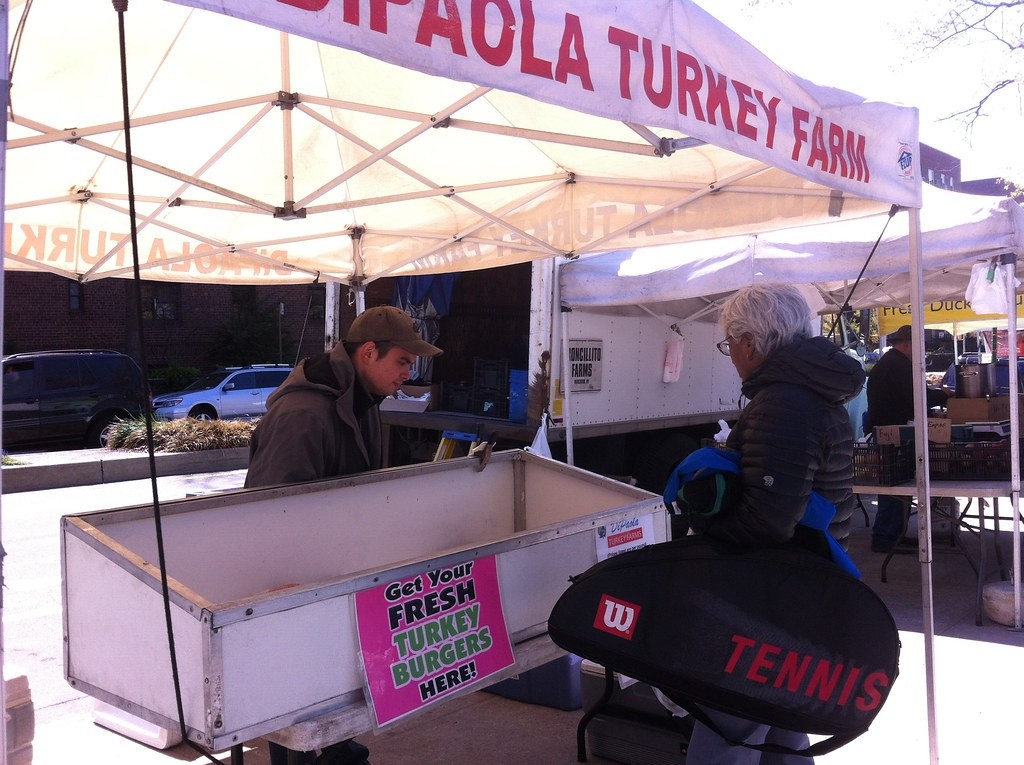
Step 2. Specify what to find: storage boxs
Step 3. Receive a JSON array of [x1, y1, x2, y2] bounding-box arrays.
[[945, 395, 1024, 436], [378, 397, 430, 413], [924, 435, 1024, 481], [846, 440, 915, 486], [395, 381, 438, 412]]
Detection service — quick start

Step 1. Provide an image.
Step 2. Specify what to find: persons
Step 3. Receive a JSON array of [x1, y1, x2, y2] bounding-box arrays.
[[867, 326, 947, 554], [244, 304, 442, 490], [679, 284, 865, 765]]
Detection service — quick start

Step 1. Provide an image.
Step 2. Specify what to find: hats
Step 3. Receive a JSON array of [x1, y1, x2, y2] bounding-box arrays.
[[888, 325, 930, 355], [346, 304, 444, 356]]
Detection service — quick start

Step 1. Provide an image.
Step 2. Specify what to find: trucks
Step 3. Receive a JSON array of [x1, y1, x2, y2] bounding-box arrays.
[[325, 237, 822, 457]]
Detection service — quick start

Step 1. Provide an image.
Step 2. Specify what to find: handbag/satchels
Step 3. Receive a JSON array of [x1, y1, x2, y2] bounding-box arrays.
[[547, 533, 901, 757]]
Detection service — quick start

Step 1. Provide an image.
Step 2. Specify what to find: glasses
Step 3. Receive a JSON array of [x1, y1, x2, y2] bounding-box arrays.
[[717, 335, 733, 357]]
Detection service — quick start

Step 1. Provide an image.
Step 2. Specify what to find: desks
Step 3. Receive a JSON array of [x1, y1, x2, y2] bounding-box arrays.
[[852, 478, 1023, 626]]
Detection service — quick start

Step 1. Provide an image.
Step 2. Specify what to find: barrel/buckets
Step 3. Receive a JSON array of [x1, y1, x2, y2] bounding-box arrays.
[[954, 362, 997, 398], [508, 369, 528, 424], [930, 497, 960, 543]]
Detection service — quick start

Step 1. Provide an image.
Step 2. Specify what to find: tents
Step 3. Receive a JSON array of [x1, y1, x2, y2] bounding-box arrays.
[[560, 177, 1023, 630], [0, 1, 937, 764]]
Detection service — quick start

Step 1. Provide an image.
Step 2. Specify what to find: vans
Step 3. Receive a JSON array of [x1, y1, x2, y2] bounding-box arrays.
[[2, 350, 152, 449]]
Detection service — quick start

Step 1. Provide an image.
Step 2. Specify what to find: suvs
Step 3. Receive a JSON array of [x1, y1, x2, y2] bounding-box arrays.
[[152, 362, 293, 421]]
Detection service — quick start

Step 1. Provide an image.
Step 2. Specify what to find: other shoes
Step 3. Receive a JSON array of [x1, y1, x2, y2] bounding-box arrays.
[[328, 741, 369, 763], [871, 536, 918, 552]]
